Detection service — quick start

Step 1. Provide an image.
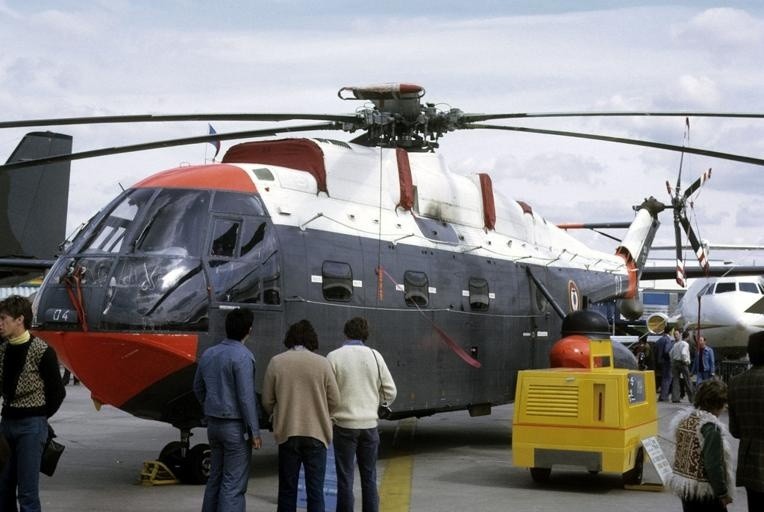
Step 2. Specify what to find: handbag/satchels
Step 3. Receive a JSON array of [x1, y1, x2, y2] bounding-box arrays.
[[378, 403, 392, 420], [39, 424, 65, 477]]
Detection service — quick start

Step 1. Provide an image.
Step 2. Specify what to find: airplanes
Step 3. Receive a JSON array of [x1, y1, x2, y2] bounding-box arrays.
[[0, 128, 75, 289], [609, 261, 764, 382]]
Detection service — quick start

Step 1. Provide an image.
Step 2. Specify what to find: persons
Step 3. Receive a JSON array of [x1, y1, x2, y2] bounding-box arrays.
[[636, 326, 715, 402], [0, 294, 66, 512], [326, 317, 397, 512], [262, 319, 341, 512], [192, 306, 263, 512], [728, 331, 764, 512], [62, 369, 79, 386], [669, 380, 733, 512]]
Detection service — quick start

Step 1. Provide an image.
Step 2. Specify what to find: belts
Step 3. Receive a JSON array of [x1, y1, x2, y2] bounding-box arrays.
[[209, 415, 243, 423], [675, 360, 682, 362]]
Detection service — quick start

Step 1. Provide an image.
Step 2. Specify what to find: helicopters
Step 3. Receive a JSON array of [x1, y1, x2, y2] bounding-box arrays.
[[0, 81, 764, 489]]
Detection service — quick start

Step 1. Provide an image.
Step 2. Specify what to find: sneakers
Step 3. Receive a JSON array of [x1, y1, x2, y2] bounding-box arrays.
[[671, 400, 680, 403], [658, 397, 669, 401]]
